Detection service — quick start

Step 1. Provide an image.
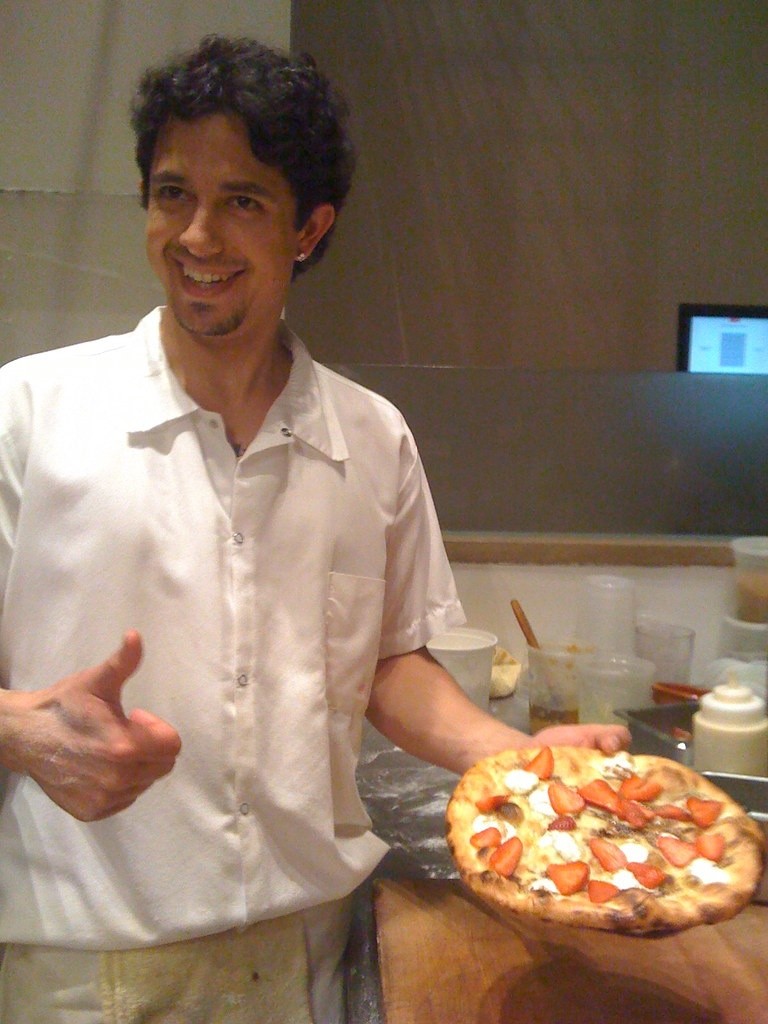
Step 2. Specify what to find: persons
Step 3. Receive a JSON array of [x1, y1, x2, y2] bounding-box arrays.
[[0, 32, 633, 1024]]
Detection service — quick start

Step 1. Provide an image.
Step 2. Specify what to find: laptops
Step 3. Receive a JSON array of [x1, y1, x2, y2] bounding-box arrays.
[[676, 303, 768, 376]]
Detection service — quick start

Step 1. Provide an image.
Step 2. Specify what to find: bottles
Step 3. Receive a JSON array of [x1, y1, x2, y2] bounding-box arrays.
[[692, 672, 768, 777]]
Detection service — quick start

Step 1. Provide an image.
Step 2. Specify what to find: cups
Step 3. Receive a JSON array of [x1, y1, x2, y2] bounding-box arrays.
[[425, 627, 498, 712], [730, 534, 768, 623], [572, 572, 695, 685], [529, 640, 596, 734], [574, 657, 657, 725]]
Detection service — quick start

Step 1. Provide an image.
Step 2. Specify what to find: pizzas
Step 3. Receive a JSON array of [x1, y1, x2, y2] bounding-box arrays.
[[445, 744, 765, 933]]
